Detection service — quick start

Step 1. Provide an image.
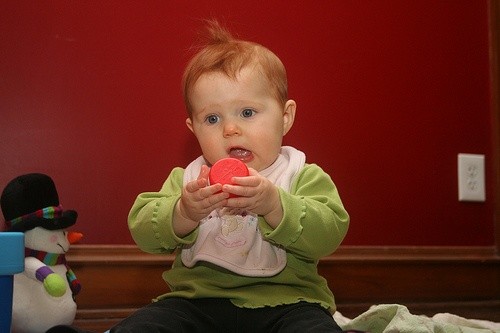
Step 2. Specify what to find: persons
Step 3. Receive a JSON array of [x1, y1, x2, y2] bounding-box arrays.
[[98, 36, 350, 332]]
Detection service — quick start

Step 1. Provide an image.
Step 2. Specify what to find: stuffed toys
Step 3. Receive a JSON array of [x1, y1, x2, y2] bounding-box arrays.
[[1, 172, 82, 333]]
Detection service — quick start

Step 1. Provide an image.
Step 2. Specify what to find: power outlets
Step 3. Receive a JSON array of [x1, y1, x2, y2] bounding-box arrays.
[[457, 153, 486, 201]]
